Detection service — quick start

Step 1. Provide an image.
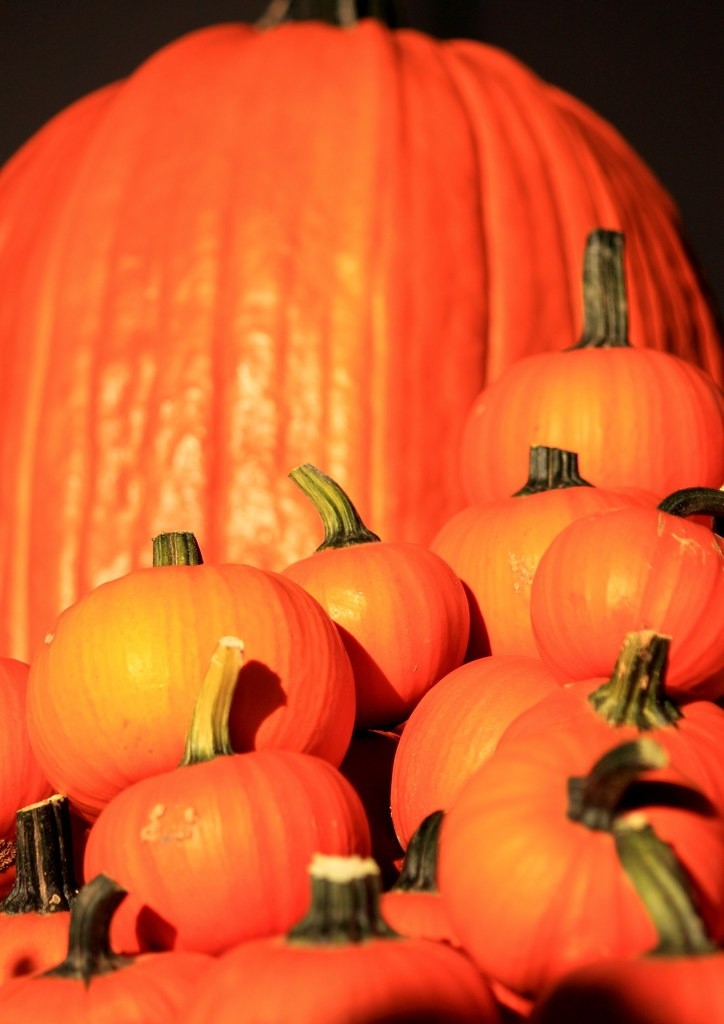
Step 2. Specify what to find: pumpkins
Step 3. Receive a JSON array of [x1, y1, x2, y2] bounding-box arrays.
[[1, 0, 724, 1024]]
